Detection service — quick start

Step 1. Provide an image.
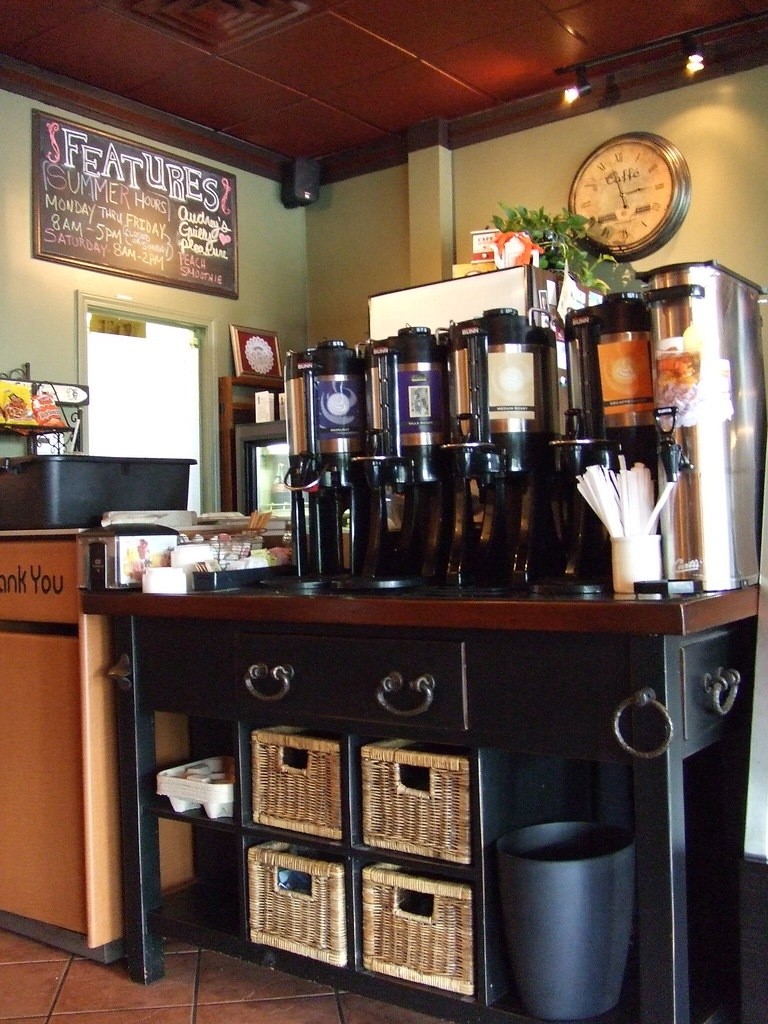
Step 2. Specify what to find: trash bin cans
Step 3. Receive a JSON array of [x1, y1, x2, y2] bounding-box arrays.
[[498, 821, 636, 1018]]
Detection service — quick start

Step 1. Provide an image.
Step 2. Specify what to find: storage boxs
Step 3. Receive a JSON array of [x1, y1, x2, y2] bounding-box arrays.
[[451, 262, 497, 278], [471, 229, 501, 262], [0, 455, 197, 529]]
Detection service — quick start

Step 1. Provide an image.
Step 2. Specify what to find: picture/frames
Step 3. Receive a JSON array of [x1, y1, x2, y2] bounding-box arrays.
[[228, 324, 284, 382]]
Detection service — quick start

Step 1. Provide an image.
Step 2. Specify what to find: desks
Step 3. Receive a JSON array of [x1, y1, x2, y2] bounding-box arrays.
[[81, 580, 759, 1024]]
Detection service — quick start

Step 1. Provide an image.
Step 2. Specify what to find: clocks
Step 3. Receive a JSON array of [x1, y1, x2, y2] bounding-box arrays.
[[568, 132, 693, 264]]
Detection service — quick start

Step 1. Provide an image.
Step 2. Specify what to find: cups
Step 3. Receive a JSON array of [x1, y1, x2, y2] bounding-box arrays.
[[611, 536, 662, 595]]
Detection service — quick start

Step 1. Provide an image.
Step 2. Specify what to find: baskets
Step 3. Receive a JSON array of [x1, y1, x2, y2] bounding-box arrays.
[[251, 725, 343, 839], [248, 840, 348, 967], [360, 738, 471, 864], [361, 863, 475, 995]]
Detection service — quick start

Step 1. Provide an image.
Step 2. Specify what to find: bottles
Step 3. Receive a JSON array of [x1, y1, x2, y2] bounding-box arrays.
[[272, 462, 290, 508]]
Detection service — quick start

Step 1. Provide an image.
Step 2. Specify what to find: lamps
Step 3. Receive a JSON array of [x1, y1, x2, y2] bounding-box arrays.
[[563, 67, 592, 105], [682, 38, 704, 64]]
